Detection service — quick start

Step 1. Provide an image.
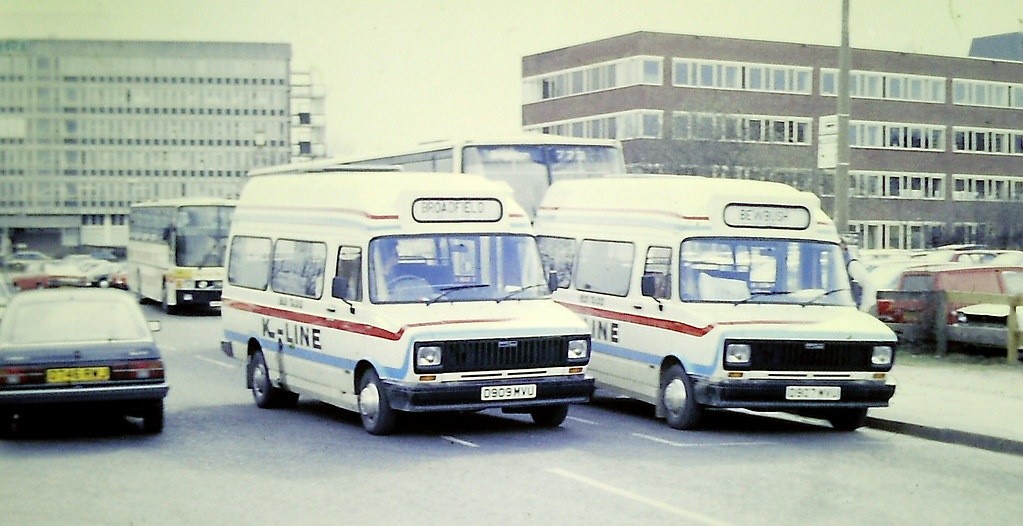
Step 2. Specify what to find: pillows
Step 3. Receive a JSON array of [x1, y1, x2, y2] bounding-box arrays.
[[697, 273, 751, 301], [788, 289, 823, 298]]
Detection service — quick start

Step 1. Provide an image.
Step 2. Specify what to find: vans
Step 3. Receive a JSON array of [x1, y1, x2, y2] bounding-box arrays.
[[220, 162, 596, 435], [533, 174, 898, 431]]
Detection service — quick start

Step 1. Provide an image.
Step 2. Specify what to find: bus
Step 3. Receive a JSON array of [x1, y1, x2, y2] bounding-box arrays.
[[126, 197, 239, 314], [340, 133, 627, 223]]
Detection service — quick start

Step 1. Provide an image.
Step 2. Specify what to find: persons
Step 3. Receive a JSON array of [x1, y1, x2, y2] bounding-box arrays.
[[382, 238, 398, 285]]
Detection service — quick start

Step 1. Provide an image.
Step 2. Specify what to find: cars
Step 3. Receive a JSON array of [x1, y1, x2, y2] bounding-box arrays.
[[3, 250, 130, 292], [0, 287, 169, 439], [859, 244, 1023, 324]]
